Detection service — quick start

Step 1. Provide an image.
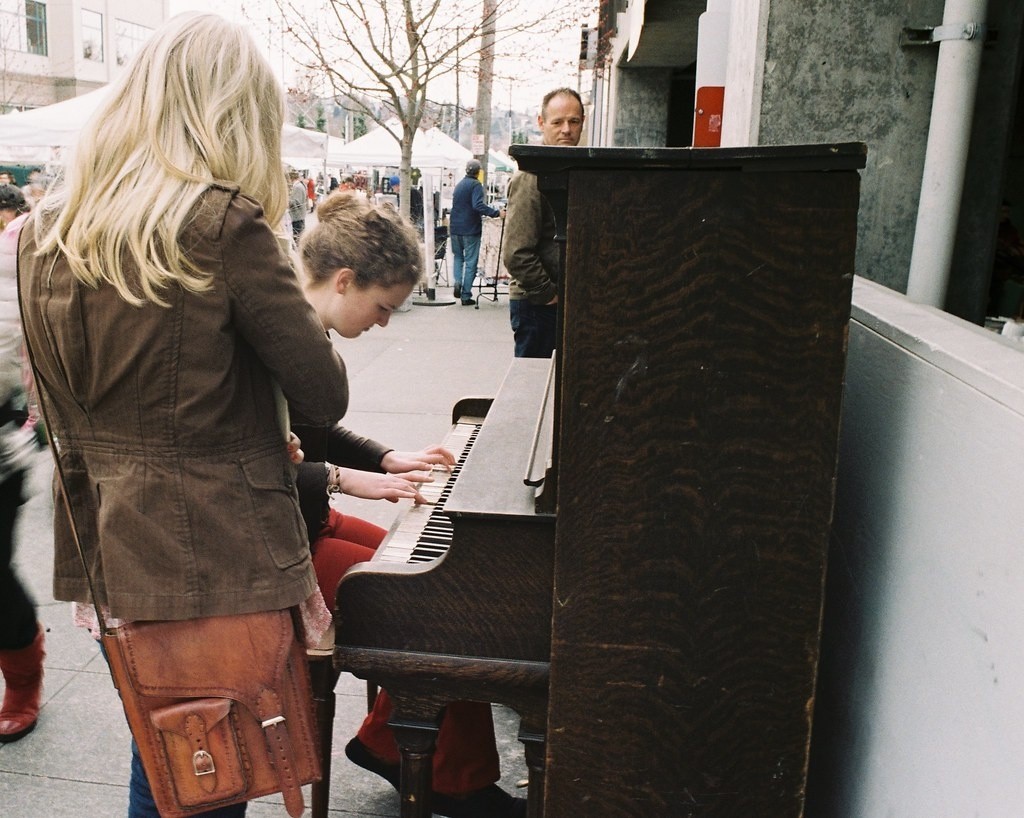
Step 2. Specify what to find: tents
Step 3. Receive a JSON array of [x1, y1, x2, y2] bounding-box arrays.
[[1, 82, 521, 189]]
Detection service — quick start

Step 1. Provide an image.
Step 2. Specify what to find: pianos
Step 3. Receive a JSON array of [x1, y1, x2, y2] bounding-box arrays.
[[334, 140, 865, 818]]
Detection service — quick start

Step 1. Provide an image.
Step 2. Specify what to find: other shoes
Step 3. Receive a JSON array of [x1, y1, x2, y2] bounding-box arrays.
[[462, 300, 476, 306], [346, 735, 401, 794], [454, 290, 462, 297], [430, 783, 528, 818]]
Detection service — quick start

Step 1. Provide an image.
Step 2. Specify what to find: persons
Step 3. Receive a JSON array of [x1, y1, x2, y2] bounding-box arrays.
[[501, 85, 585, 357], [449, 161, 508, 306], [13, 11, 353, 818], [284, 166, 456, 303], [277, 187, 538, 818], [0, 154, 68, 747]]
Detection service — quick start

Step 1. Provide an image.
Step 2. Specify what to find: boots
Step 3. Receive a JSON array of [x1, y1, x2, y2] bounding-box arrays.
[[0, 622, 44, 744]]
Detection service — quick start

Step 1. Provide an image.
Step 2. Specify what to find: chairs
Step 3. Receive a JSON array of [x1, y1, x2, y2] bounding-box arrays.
[[434, 225, 449, 288]]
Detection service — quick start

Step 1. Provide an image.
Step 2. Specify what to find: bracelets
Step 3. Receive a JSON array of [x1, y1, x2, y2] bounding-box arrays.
[[325, 464, 340, 500]]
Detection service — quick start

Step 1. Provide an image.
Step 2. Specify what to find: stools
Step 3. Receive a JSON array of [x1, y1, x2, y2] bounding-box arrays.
[[307, 616, 378, 818]]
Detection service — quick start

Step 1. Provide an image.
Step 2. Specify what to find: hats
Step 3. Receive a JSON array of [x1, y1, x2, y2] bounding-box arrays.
[[0, 184, 26, 209], [386, 177, 400, 190], [466, 159, 483, 171]]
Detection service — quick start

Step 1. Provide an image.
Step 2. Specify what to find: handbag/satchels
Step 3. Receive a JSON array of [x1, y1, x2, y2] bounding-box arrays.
[[100, 608, 322, 818]]
